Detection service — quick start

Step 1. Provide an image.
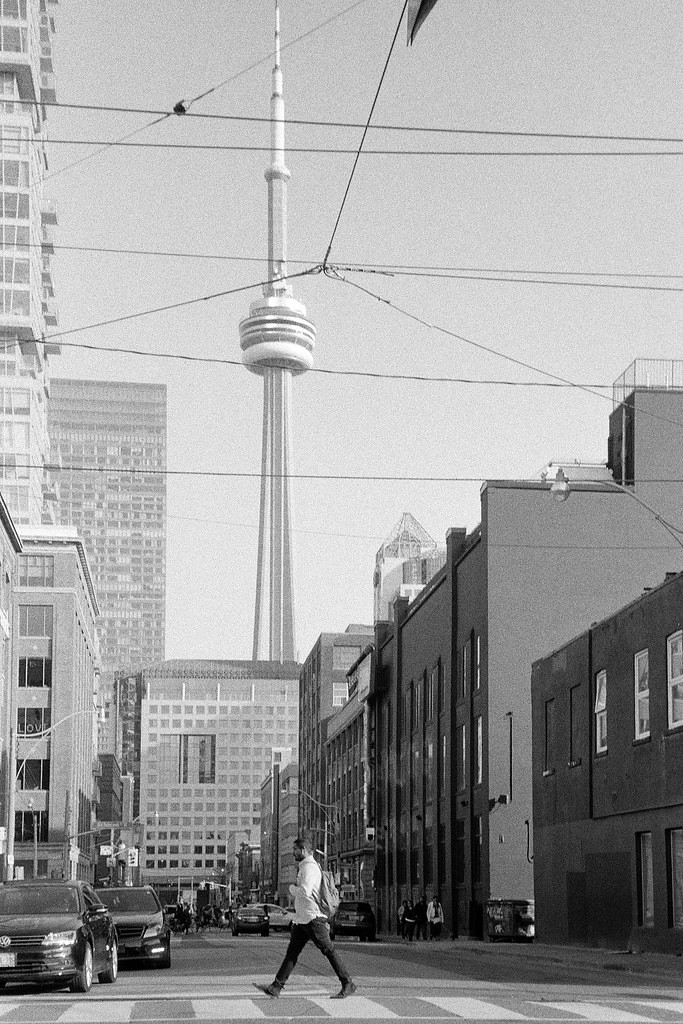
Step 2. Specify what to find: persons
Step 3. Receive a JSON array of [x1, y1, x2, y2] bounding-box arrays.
[[175, 901, 235, 936], [252, 836, 358, 999], [397, 896, 447, 941]]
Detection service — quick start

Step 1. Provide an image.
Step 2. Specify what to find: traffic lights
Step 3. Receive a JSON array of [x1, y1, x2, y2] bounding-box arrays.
[[128, 849, 139, 867]]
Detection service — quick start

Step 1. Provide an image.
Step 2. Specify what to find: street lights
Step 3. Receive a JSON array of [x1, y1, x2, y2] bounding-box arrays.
[[5, 706, 107, 880], [26, 798, 38, 880], [279, 786, 338, 872], [109, 809, 160, 888]]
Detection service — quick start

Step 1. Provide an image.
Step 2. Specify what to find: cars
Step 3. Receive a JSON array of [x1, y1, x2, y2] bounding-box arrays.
[[0, 879, 120, 993], [230, 908, 270, 937], [93, 885, 178, 969], [245, 903, 296, 933], [329, 901, 377, 942]]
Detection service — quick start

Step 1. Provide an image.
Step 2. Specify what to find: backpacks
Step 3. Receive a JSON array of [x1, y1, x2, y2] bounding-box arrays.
[[302, 861, 340, 916]]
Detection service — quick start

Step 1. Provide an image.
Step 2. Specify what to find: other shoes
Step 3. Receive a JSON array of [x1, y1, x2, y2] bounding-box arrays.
[[253, 983, 278, 998], [330, 978, 357, 999]]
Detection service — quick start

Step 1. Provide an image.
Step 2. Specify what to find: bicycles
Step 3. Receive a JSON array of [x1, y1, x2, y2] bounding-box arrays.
[[167, 908, 226, 935]]
